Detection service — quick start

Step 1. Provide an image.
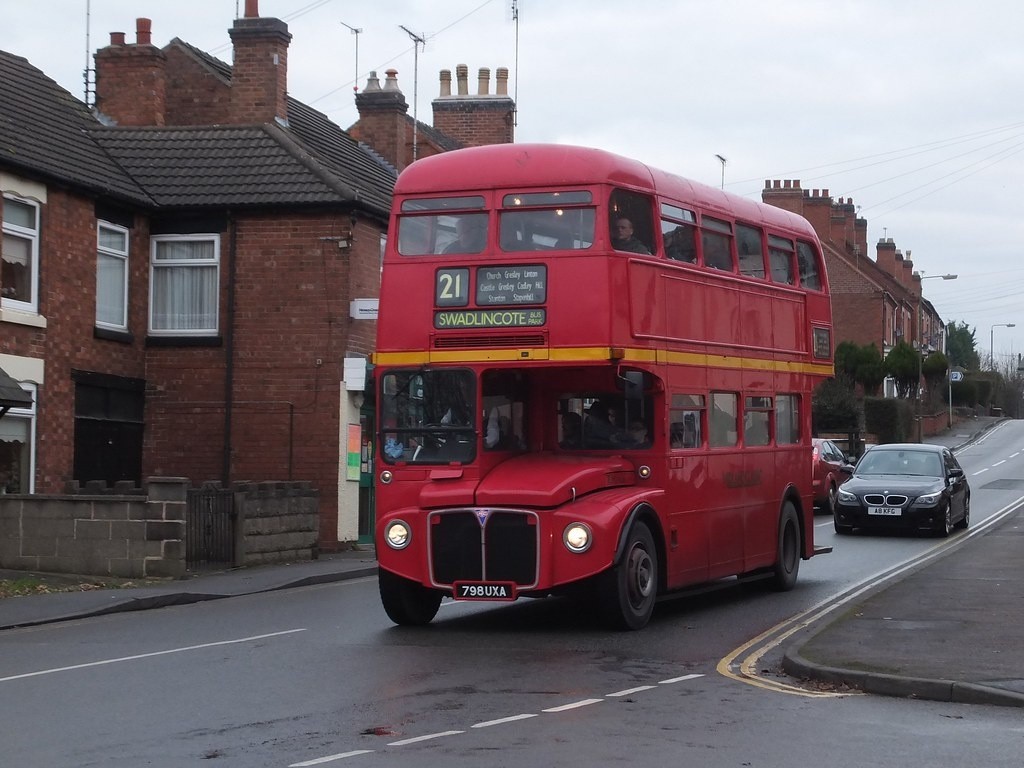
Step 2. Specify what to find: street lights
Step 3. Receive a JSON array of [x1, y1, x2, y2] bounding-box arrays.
[[917, 274, 958, 444], [990, 324, 1015, 416]]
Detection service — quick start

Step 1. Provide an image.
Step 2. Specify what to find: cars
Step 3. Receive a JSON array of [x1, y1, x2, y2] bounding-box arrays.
[[991, 408, 1007, 417], [834, 443, 970, 536], [811, 438, 858, 514]]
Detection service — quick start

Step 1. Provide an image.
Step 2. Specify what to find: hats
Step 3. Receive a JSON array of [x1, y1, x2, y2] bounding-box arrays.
[[584, 401, 609, 417]]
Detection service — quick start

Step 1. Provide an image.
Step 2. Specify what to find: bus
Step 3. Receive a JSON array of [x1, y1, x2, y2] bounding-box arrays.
[[368, 143, 839, 628]]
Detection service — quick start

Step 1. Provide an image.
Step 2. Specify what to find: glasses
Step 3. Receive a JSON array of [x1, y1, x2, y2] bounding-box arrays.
[[630, 427, 645, 431]]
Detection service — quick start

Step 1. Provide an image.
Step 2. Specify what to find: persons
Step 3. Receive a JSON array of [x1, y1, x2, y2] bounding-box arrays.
[[612, 217, 685, 261], [439, 377, 500, 452], [560, 401, 650, 449], [442, 219, 487, 255]]
